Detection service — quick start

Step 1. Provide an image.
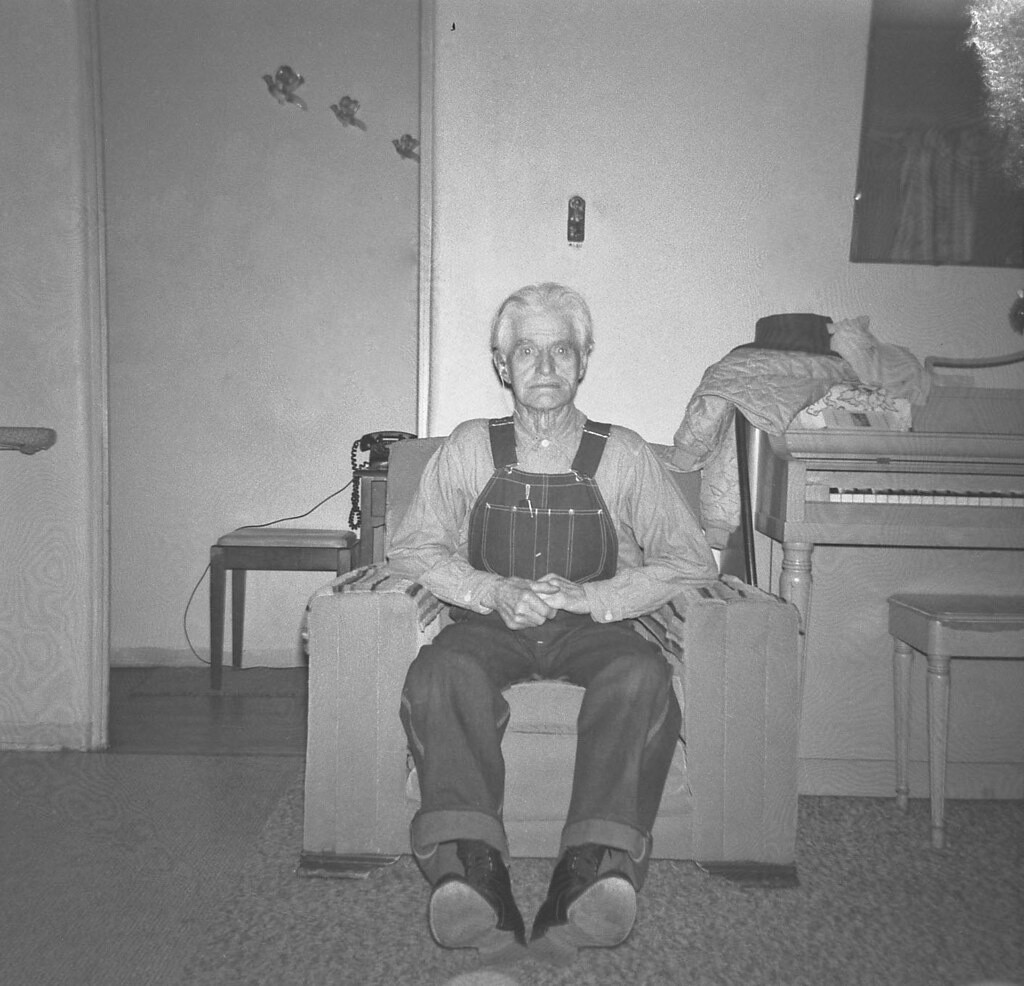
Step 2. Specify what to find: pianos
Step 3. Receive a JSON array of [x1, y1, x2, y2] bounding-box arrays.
[[753, 386, 1024, 701]]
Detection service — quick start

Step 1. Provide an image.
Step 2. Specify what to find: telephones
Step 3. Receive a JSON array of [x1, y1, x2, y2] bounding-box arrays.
[[360, 431, 419, 472]]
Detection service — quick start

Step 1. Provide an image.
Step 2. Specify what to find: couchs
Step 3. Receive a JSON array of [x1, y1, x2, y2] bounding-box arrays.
[[296, 431, 802, 886]]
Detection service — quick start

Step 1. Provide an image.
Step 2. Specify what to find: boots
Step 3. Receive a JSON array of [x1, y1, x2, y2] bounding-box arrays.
[[527, 841, 638, 958], [429, 839, 525, 959]]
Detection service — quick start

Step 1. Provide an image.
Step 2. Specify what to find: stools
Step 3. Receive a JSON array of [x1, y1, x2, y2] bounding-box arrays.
[[885, 589, 1023, 849], [207, 525, 361, 693]]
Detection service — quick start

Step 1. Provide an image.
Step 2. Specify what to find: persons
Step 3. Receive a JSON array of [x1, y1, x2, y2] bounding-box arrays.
[[398, 277, 722, 961]]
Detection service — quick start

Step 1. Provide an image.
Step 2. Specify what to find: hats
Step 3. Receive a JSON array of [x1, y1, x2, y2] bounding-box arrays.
[[734, 312, 844, 357]]
